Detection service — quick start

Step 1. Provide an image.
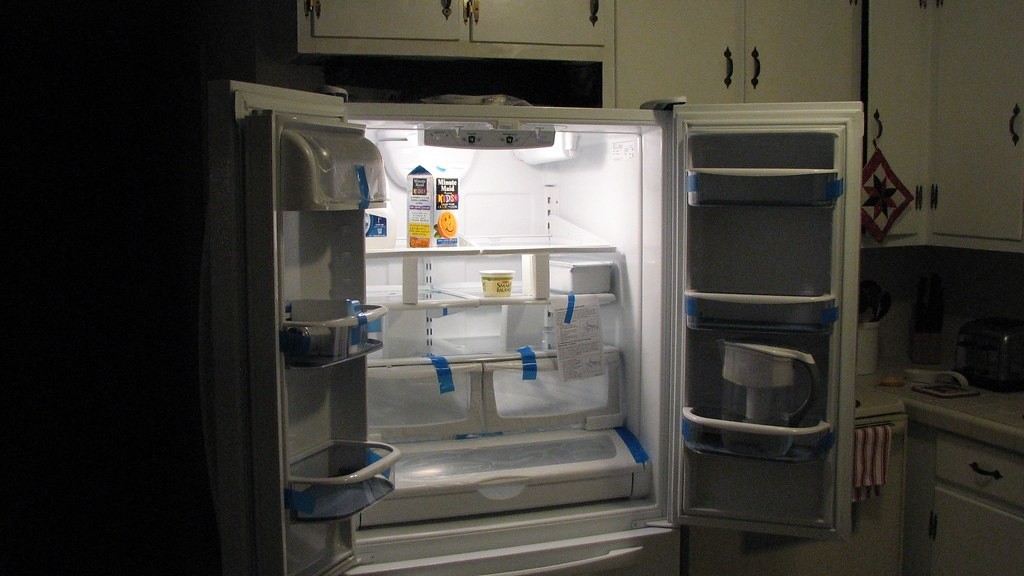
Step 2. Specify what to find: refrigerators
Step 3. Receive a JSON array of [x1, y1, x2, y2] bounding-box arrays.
[[197, 78, 864, 576]]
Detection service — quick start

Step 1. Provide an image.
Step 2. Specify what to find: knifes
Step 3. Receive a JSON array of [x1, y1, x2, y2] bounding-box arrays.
[[912, 271, 943, 333]]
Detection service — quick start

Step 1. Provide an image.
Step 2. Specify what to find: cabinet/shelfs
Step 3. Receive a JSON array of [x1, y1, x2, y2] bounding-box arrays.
[[682, 357, 1024, 576], [601, 1, 859, 110], [288, 0, 611, 62], [922, 0, 1024, 255], [852, 0, 929, 248]]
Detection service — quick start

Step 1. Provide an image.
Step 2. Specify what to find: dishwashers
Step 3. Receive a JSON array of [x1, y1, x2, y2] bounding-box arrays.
[[687, 413, 909, 576]]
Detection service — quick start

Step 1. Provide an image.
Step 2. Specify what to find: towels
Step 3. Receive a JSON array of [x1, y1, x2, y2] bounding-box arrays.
[[852, 425, 892, 505]]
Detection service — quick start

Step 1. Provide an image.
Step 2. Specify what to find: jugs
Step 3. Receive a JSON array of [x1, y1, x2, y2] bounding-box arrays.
[[716, 338, 819, 457]]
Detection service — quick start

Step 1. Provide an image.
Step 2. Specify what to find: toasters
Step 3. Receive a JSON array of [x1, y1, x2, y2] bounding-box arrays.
[[955, 318, 1024, 393]]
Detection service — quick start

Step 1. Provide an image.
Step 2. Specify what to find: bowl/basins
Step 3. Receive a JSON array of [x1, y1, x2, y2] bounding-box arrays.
[[479, 270, 515, 297], [903, 368, 935, 383]]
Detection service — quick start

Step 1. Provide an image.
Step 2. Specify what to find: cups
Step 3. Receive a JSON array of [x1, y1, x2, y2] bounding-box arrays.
[[858, 321, 880, 376]]
